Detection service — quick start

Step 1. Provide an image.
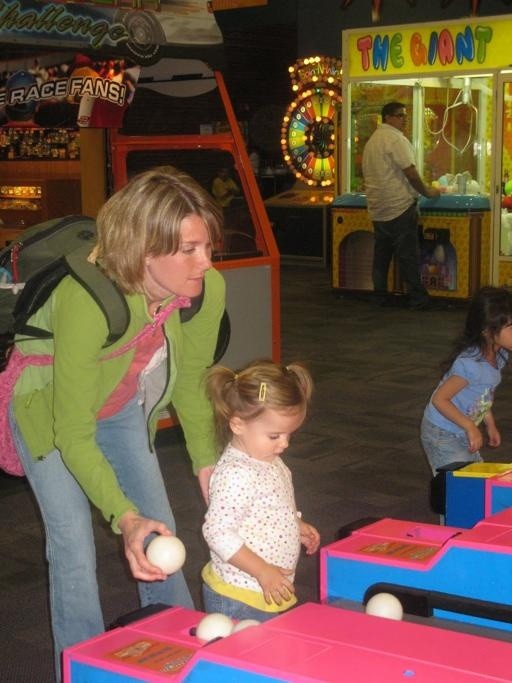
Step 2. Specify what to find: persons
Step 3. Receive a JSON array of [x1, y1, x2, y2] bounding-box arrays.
[[212, 164, 243, 216], [362, 101, 445, 311], [418, 285, 511, 527], [197, 357, 322, 621], [0, 164, 229, 683]]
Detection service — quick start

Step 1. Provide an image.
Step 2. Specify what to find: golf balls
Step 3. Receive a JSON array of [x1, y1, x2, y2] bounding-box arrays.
[[196, 614, 261, 642], [366, 593, 403, 620], [146, 535, 186, 575]]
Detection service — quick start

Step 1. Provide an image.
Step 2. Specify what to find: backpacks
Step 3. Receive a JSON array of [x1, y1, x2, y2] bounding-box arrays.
[[0, 214, 130, 371]]
